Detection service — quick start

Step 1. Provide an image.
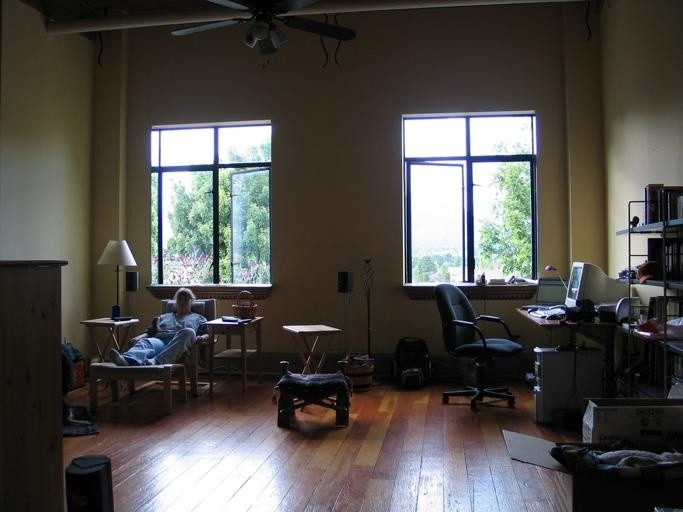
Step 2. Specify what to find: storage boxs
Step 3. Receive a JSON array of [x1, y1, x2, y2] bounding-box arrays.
[[502, 398, 683, 512]]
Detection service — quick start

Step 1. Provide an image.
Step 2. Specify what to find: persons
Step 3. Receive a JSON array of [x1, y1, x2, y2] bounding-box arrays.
[[109, 288, 209, 366]]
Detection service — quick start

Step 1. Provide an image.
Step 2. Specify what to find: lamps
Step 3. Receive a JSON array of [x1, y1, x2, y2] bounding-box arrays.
[[97, 240, 137, 320], [545, 265, 568, 291], [239, 3, 289, 49]]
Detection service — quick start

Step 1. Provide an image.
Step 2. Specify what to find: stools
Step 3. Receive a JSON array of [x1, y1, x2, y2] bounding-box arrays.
[[277, 372, 352, 428], [88, 362, 188, 415]]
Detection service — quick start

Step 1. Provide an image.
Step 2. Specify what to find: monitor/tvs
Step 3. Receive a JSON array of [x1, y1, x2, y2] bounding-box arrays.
[[564, 262, 629, 307]]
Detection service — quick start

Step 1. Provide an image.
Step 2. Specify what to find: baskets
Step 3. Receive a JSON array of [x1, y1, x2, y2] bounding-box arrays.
[[231, 291, 257, 319]]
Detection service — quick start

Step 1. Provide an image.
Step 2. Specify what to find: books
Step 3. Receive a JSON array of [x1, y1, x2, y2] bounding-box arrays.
[[644, 182, 682, 326]]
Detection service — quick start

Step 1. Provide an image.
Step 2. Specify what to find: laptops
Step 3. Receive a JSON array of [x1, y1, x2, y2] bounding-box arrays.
[[520, 276, 569, 310]]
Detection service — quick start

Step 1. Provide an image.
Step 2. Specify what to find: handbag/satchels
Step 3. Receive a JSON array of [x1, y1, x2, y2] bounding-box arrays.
[[393, 337, 434, 388]]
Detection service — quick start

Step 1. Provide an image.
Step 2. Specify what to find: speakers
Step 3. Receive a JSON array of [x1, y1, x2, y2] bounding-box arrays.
[[338, 271, 353, 293], [126, 272, 139, 292]]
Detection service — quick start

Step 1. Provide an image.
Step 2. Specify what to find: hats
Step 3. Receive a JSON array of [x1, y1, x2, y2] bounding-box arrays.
[[637, 261, 659, 284]]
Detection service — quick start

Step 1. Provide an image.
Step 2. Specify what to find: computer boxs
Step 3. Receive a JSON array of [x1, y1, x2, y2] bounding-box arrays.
[[530, 344, 606, 426]]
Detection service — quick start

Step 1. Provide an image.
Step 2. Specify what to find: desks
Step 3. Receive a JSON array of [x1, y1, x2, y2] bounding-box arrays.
[[283, 325, 342, 413], [514, 306, 622, 394], [80, 318, 139, 391], [201, 316, 263, 400]]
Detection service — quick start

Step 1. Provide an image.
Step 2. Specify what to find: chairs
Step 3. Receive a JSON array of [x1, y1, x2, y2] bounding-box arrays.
[[433, 283, 523, 413], [127, 299, 216, 397]]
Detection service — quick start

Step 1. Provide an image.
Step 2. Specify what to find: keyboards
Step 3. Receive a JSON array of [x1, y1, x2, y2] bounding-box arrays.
[[529, 308, 565, 318]]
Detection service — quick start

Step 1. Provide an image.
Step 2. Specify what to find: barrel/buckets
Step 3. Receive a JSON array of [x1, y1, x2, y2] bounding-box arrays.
[[344, 362, 374, 393]]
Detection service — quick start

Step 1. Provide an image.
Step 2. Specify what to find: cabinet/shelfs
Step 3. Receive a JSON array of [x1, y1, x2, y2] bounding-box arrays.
[[615, 201, 683, 396]]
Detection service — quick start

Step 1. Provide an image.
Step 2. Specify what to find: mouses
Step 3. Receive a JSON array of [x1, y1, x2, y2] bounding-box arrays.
[[546, 314, 562, 320]]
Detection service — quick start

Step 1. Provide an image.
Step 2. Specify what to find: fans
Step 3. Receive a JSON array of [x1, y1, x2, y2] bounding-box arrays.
[[169, 0, 357, 55]]
